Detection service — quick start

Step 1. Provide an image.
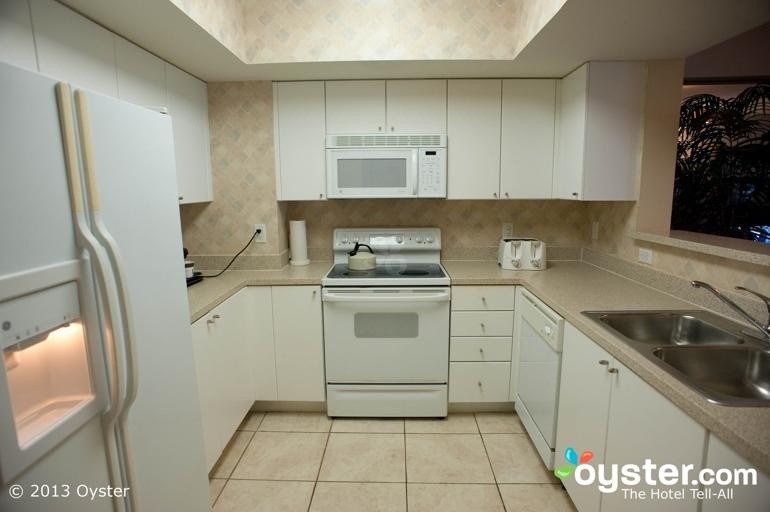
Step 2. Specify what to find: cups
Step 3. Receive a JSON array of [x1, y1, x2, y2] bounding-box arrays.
[[184, 261, 196, 278]]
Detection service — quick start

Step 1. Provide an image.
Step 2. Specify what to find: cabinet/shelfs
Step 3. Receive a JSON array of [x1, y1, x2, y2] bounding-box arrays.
[[699, 432, 769, 510], [250, 282, 327, 417], [162, 59, 214, 207], [112, 31, 167, 117], [268, 56, 649, 205], [28, 1, 116, 97], [446, 280, 516, 417], [551, 321, 706, 510], [190, 285, 252, 485], [0, 0, 37, 74]]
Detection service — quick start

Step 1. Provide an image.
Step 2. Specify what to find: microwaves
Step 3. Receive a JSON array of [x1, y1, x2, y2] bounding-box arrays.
[[325, 132, 447, 197]]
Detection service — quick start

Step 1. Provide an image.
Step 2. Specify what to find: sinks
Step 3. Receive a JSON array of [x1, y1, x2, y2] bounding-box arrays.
[[637, 343, 770, 408], [579, 310, 763, 348]]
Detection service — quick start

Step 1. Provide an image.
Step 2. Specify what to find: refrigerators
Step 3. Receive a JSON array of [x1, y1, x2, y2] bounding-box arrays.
[[0, 60, 215, 512]]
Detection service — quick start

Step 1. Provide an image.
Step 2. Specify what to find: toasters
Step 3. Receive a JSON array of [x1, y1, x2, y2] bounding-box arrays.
[[497, 238, 547, 272]]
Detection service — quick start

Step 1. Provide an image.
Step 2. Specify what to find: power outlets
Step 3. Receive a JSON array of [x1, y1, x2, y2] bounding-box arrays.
[[254, 222, 268, 245]]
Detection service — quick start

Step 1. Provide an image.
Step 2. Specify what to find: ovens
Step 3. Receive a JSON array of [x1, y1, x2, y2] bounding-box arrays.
[[321, 287, 449, 420]]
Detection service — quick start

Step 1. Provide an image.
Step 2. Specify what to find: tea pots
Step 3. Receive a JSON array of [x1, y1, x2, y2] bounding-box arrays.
[[348, 242, 377, 270]]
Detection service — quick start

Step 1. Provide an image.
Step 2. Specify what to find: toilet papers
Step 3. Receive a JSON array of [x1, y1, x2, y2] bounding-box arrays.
[[288, 218, 307, 262]]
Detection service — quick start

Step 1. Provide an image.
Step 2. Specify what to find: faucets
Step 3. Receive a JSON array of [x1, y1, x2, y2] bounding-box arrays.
[[690, 279, 770, 339]]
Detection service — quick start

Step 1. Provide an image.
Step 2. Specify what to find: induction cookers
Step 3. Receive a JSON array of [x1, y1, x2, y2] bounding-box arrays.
[[321, 262, 451, 286]]
